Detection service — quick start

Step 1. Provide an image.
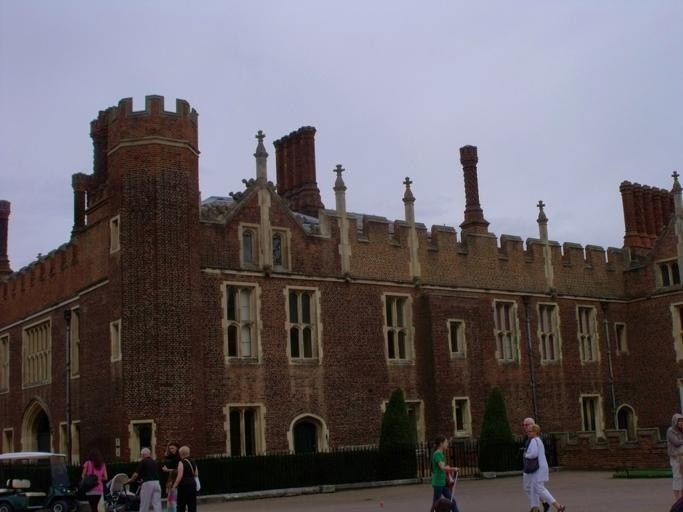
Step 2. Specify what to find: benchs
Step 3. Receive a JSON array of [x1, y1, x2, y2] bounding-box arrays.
[[0, 478, 46, 505]]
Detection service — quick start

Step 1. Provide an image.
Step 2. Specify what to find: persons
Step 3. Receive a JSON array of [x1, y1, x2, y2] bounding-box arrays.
[[427, 433, 461, 511], [521, 423, 565, 511], [170, 445, 197, 511], [519, 418, 550, 511], [432, 497, 454, 512], [665, 413, 682, 501], [121, 447, 163, 511], [160, 442, 181, 494], [80, 449, 109, 512]]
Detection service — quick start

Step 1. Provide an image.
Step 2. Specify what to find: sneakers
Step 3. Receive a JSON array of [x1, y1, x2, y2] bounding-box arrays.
[[525, 500, 567, 512]]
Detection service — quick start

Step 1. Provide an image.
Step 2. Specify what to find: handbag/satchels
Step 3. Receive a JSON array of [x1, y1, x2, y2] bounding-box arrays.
[[193, 474, 202, 493], [82, 462, 99, 494], [522, 438, 541, 474]]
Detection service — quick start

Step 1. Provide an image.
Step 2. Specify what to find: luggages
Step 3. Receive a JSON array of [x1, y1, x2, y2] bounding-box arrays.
[[433, 470, 459, 511]]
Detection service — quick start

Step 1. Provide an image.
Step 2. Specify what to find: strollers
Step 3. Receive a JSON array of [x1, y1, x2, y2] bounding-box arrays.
[[103, 473, 144, 512]]
